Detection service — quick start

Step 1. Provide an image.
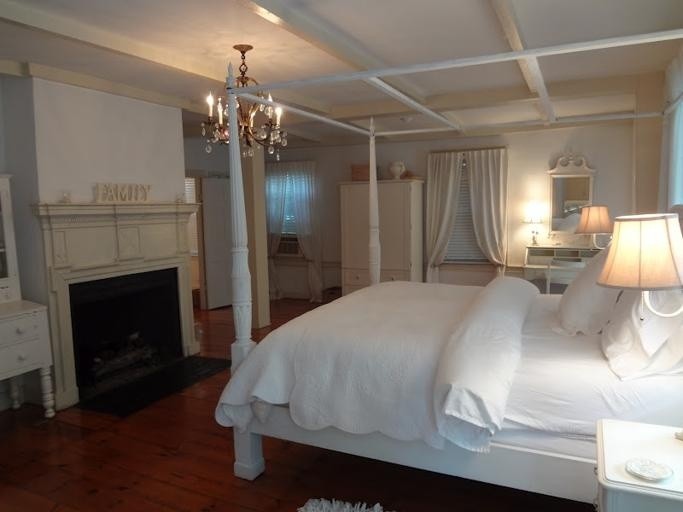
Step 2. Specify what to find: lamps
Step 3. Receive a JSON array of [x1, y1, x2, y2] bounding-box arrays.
[[598, 212, 683, 318], [574, 205, 612, 250], [203, 46, 287, 164]]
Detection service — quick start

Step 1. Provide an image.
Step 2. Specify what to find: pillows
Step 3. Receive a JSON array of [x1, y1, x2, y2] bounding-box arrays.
[[558, 250, 620, 334], [601, 288, 683, 380]]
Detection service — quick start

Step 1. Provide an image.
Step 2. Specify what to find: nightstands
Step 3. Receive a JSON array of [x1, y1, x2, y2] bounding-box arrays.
[[596, 419, 683, 512]]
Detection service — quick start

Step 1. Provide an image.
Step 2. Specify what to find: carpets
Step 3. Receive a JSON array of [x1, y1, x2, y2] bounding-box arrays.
[[80, 355, 232, 418]]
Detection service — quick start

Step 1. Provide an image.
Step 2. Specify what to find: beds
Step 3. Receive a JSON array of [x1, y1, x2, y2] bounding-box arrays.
[[214, 206, 683, 503]]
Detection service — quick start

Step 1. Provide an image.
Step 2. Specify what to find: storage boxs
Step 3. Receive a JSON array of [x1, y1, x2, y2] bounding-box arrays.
[[351, 164, 369, 182], [322, 287, 341, 303]]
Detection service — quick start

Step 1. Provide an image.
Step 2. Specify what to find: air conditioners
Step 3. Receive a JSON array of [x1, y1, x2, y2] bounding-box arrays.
[[271, 232, 304, 257]]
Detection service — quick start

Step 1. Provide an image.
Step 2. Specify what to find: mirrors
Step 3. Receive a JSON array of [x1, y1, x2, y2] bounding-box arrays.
[[546, 156, 599, 235]]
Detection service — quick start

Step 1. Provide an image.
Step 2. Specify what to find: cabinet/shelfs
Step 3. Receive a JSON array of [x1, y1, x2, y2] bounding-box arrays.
[[338, 178, 424, 296], [0, 300, 54, 418]]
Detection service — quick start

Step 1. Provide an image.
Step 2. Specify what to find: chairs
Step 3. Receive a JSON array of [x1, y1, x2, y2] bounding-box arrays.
[[544, 257, 585, 294]]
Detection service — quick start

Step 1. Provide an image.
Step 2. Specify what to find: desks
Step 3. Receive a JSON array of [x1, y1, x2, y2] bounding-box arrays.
[[522, 243, 607, 279]]
